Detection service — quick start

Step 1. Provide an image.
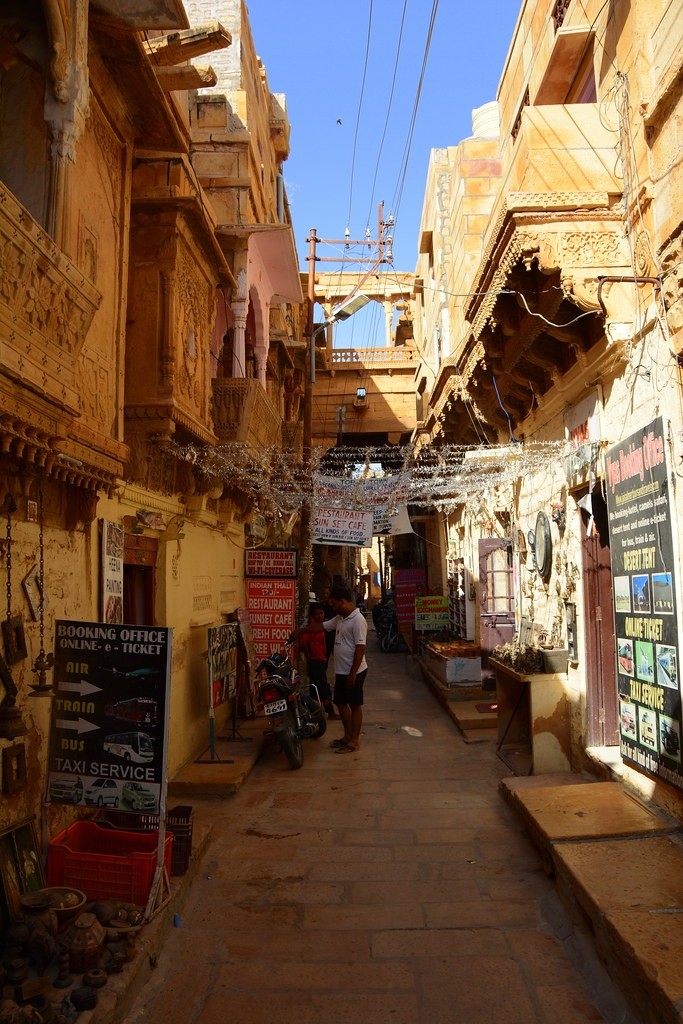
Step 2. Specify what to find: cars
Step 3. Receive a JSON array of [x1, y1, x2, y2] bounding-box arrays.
[[83, 777, 120, 809], [121, 781, 158, 811]]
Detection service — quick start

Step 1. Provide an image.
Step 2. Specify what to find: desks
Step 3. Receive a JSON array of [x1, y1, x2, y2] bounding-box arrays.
[[487, 657, 570, 777]]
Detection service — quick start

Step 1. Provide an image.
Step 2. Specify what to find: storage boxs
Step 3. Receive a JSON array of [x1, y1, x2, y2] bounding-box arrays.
[[46, 821, 174, 907], [92, 806, 194, 876]]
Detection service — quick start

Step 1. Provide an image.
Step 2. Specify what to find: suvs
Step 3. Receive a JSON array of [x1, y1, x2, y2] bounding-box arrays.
[[49, 773, 84, 805]]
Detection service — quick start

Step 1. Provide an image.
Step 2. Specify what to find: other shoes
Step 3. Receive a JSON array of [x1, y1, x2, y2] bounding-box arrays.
[[327, 713, 341, 720]]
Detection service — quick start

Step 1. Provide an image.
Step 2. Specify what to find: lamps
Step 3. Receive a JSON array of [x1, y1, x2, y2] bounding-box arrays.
[[352, 388, 369, 408], [137, 510, 154, 526]]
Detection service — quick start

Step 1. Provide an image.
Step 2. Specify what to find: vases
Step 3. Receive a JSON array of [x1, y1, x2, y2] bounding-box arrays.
[[19, 892, 61, 950]]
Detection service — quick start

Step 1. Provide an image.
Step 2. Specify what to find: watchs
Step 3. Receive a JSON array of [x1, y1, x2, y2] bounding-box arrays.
[[301, 627, 305, 632]]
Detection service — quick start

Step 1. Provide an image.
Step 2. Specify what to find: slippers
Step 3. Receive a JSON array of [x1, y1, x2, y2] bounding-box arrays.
[[330, 738, 347, 747], [334, 744, 359, 754]]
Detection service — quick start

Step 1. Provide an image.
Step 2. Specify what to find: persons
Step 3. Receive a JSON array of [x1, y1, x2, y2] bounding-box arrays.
[[289, 586, 368, 754]]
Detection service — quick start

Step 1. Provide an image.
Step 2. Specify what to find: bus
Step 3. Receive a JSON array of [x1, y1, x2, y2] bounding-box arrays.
[[103, 698, 156, 730], [93, 731, 156, 764]]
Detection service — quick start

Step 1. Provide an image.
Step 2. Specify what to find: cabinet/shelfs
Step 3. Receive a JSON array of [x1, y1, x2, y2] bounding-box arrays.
[[447, 557, 475, 641]]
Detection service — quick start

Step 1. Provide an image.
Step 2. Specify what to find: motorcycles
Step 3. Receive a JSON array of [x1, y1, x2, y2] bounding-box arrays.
[[373, 607, 399, 653], [252, 631, 328, 770]]
